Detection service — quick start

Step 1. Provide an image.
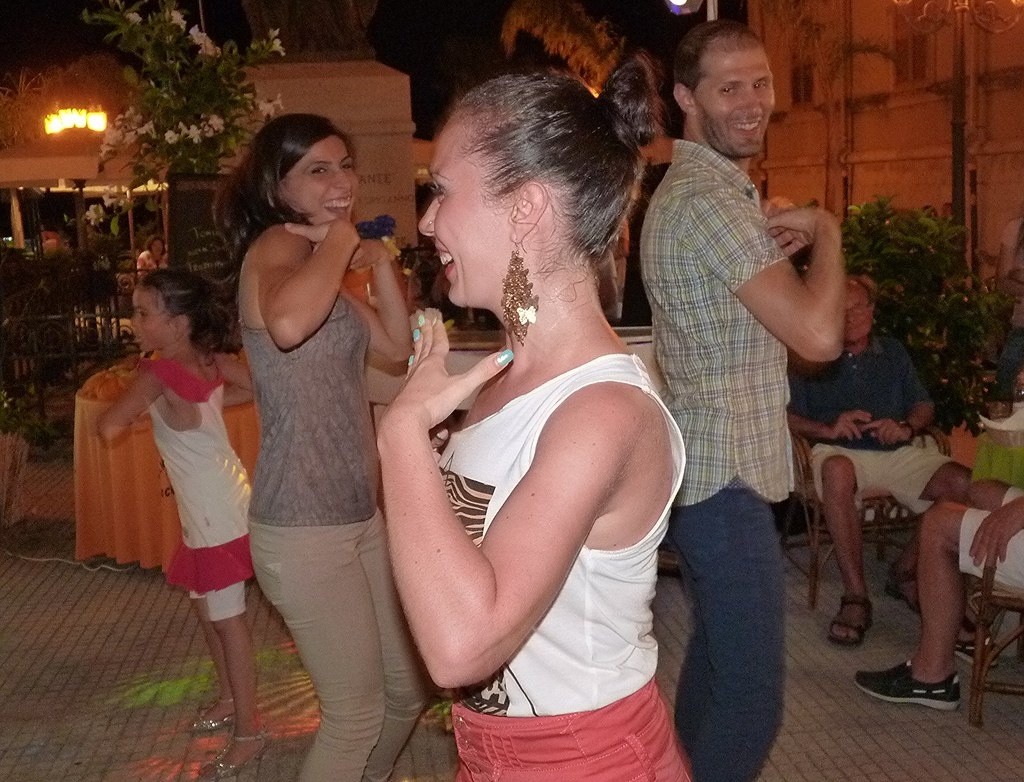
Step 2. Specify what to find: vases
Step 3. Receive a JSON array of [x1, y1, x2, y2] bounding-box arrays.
[[166, 173, 246, 353]]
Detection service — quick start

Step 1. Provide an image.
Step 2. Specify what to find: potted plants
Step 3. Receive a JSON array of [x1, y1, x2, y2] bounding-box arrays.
[[799, 191, 1023, 521]]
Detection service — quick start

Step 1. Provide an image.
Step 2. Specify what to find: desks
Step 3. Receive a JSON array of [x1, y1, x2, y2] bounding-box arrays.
[[71, 355, 258, 575]]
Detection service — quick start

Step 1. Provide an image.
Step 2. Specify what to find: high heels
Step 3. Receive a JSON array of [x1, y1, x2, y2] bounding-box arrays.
[[190, 696, 236, 732], [201, 725, 273, 780]]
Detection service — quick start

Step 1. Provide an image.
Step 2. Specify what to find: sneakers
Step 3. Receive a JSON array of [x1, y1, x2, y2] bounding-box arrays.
[[855, 658, 961, 712], [956, 635, 998, 668]]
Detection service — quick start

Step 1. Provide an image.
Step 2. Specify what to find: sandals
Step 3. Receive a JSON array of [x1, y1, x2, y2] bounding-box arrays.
[[828, 593, 872, 646], [884, 561, 921, 613]]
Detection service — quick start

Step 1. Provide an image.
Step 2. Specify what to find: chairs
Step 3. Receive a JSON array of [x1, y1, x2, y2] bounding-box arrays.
[[779, 421, 953, 610], [968, 555, 1024, 726]]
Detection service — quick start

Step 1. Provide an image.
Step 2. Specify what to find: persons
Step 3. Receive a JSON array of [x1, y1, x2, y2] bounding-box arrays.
[[99, 267, 271, 782], [644, 20, 848, 781], [787, 272, 972, 646], [136, 234, 167, 276], [855, 479, 1023, 711], [592, 220, 630, 327], [0, 248, 70, 418], [375, 59, 694, 782], [214, 111, 429, 782]]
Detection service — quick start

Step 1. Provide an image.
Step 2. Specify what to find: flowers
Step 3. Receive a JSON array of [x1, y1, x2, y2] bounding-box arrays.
[[78, 0, 294, 238]]
